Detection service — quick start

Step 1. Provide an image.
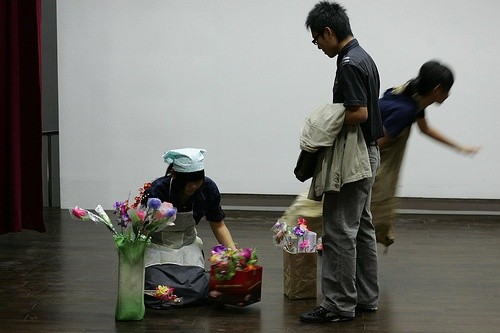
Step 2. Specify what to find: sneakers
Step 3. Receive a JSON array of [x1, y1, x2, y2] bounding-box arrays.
[[354, 304, 378, 313], [298, 305, 354, 323]]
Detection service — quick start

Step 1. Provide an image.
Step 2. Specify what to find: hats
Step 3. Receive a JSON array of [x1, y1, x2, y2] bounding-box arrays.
[[162, 148, 207, 172]]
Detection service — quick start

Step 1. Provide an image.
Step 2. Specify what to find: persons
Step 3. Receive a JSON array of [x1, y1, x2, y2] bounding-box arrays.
[[299, 0, 385, 321], [141, 148, 238, 310], [370, 62, 482, 246]]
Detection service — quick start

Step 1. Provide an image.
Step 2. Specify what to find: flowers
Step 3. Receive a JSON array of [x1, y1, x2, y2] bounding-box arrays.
[[68, 182, 178, 249], [273, 218, 322, 252], [155, 284, 183, 303], [205, 242, 260, 281]]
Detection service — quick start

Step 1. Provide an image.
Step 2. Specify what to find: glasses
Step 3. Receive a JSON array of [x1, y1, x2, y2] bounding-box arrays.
[[312, 32, 321, 45]]
